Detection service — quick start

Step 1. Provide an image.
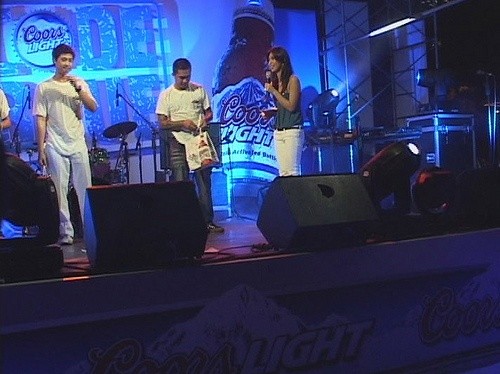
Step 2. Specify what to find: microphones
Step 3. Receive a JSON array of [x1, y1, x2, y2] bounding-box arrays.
[[28, 86, 31, 109], [266, 70, 272, 97], [116, 83, 119, 107]]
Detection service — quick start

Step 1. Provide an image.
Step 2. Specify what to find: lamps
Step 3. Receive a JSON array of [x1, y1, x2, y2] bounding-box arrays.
[[305, 88, 339, 138], [416, 66, 470, 110], [357, 141, 422, 218]]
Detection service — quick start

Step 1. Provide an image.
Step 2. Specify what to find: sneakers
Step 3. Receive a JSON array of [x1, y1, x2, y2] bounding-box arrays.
[[61, 234, 73, 245], [207, 223, 224, 233]]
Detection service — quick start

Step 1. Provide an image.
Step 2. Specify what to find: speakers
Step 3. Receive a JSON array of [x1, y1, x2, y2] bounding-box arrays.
[[451, 167, 500, 230], [255, 171, 379, 251], [82, 180, 210, 273]]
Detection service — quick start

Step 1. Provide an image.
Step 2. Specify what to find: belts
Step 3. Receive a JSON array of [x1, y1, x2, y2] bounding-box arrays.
[[278, 126, 303, 131]]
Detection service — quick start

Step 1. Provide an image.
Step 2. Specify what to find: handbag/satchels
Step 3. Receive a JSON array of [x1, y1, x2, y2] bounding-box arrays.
[[185, 128, 220, 172]]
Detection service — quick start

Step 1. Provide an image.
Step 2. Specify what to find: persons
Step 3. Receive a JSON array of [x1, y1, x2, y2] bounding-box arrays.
[[0, 88, 11, 129], [32, 44, 98, 245], [261, 47, 305, 177], [155, 58, 224, 232]]
